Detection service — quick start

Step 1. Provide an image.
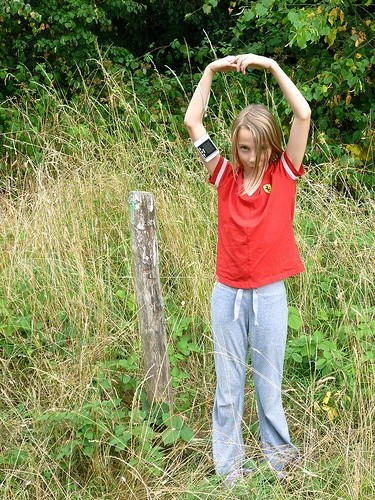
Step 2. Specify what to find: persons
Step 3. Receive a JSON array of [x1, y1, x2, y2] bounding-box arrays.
[[183, 53, 312, 489]]
